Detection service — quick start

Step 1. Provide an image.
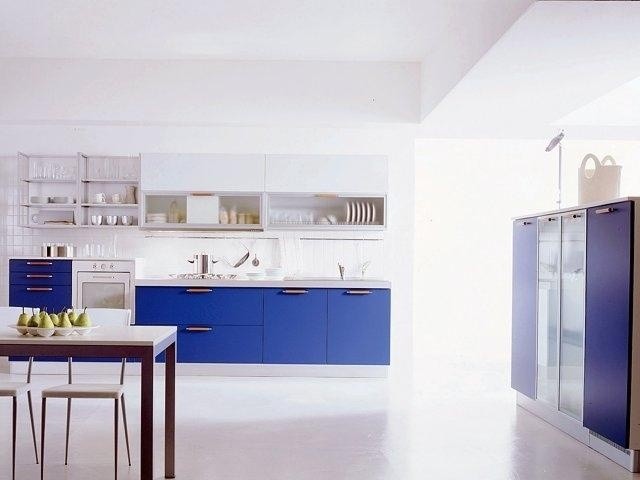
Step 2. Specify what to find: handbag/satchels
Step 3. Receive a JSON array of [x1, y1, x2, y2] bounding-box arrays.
[[577, 154, 621, 206]]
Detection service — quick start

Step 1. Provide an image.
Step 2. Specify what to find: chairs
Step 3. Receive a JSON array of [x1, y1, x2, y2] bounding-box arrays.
[[0, 308, 41, 480], [41, 306, 131, 480]]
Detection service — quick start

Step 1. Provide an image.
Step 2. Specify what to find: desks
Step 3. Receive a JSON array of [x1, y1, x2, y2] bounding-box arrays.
[[0, 324, 178, 480]]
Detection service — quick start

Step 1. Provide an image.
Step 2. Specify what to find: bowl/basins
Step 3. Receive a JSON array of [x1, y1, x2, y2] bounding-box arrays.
[[315, 214, 337, 225]]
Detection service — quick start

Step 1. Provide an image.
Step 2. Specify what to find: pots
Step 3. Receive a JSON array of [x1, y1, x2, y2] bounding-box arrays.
[[41, 242, 77, 258], [186, 252, 219, 274]]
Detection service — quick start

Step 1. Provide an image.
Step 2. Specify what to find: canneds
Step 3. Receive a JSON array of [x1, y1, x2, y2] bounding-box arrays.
[[246, 213, 254, 224], [237, 213, 246, 224]]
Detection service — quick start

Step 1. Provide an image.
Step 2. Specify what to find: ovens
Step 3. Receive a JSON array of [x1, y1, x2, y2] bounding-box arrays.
[[72, 260, 136, 325]]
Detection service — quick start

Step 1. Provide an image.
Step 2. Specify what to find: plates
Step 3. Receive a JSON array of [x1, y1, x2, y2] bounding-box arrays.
[[29, 195, 74, 203], [344, 201, 376, 224], [146, 214, 168, 224], [245, 267, 283, 280]]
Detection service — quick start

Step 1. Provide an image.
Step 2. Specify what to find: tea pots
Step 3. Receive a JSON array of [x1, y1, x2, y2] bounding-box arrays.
[[124, 186, 135, 204]]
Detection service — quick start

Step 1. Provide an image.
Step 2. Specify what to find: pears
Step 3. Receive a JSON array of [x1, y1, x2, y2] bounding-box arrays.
[[17, 305, 91, 337]]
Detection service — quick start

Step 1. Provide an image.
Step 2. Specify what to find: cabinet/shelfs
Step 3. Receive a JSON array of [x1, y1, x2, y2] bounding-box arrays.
[[8, 258, 72, 362], [264, 285, 391, 378], [140, 190, 267, 232], [507, 195, 640, 475], [18, 150, 79, 230], [135, 286, 264, 377], [266, 190, 388, 231], [79, 151, 140, 230]]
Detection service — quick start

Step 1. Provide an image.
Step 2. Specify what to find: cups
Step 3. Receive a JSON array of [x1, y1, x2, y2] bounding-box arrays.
[[83, 239, 111, 258], [33, 160, 67, 180], [219, 211, 259, 224], [94, 192, 123, 204], [271, 208, 315, 225], [92, 215, 134, 226], [168, 207, 178, 223]]
